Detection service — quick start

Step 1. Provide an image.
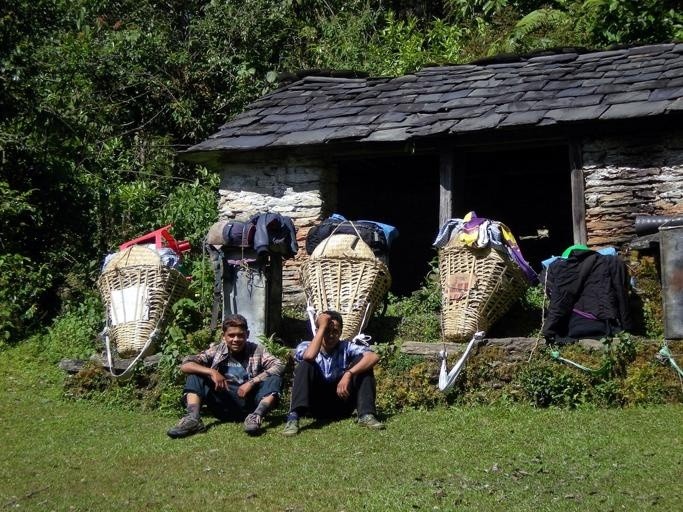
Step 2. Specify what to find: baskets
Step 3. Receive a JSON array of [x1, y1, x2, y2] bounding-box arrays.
[[297, 255, 392, 342], [436, 243, 529, 342], [97, 265, 189, 358]]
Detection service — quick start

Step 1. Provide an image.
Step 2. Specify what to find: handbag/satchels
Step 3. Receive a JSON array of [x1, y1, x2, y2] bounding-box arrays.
[[305, 218, 388, 257], [328, 213, 402, 264]]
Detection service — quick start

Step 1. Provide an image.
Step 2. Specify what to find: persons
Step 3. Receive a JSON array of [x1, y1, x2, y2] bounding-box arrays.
[[166, 314, 286, 438], [282, 310, 386, 438]]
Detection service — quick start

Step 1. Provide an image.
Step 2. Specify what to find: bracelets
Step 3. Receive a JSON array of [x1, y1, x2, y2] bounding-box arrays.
[[248, 378, 255, 385], [345, 370, 352, 374]]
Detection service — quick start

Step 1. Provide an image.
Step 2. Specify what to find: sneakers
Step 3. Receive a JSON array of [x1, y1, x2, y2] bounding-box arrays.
[[166, 416, 206, 438], [282, 415, 300, 437], [357, 414, 385, 430], [243, 413, 262, 433]]
